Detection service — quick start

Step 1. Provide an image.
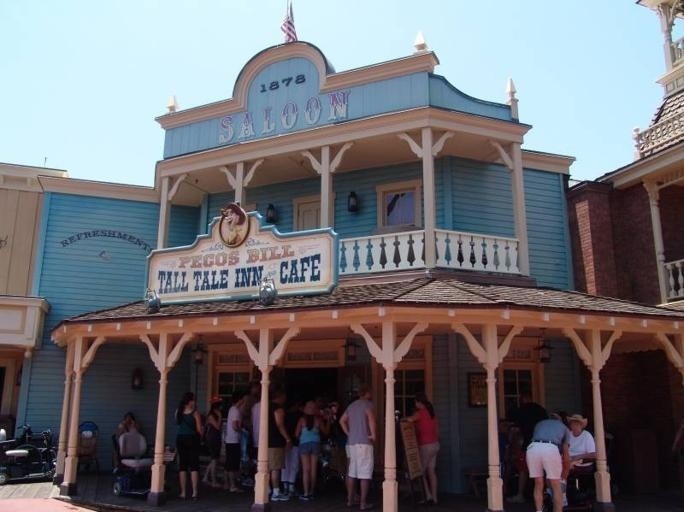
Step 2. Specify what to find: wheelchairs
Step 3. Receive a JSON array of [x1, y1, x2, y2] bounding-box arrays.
[[109, 426, 154, 498]]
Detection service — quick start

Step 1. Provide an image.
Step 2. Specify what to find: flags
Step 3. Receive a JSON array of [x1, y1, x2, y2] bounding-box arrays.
[[280, 2, 296, 45]]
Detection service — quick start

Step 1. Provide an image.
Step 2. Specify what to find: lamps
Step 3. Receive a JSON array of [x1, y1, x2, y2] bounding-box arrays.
[[265, 203, 277, 224], [342, 338, 362, 361], [348, 191, 359, 213], [535, 335, 555, 363], [131, 363, 145, 391], [192, 342, 208, 365]]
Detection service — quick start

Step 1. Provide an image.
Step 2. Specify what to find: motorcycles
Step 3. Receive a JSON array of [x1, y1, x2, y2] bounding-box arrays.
[[0, 424, 59, 485]]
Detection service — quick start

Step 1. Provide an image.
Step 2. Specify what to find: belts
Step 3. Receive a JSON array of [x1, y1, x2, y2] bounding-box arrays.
[[531, 438, 560, 447]]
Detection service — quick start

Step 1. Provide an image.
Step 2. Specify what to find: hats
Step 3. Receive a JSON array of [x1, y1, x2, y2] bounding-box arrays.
[[566, 414, 588, 426]]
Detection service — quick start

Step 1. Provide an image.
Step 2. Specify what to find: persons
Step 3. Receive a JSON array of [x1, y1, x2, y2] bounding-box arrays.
[[398, 391, 440, 505], [172, 390, 205, 502], [222, 382, 345, 503], [552, 412, 599, 479], [337, 384, 377, 510], [523, 410, 571, 511], [201, 394, 224, 489], [113, 410, 141, 439]]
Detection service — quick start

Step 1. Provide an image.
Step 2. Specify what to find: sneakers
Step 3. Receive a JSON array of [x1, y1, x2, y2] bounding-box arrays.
[[346, 496, 374, 510], [175, 495, 201, 504], [421, 498, 438, 506], [202, 478, 222, 489], [224, 483, 248, 496], [506, 494, 526, 504], [270, 487, 295, 503], [300, 495, 316, 504]]
